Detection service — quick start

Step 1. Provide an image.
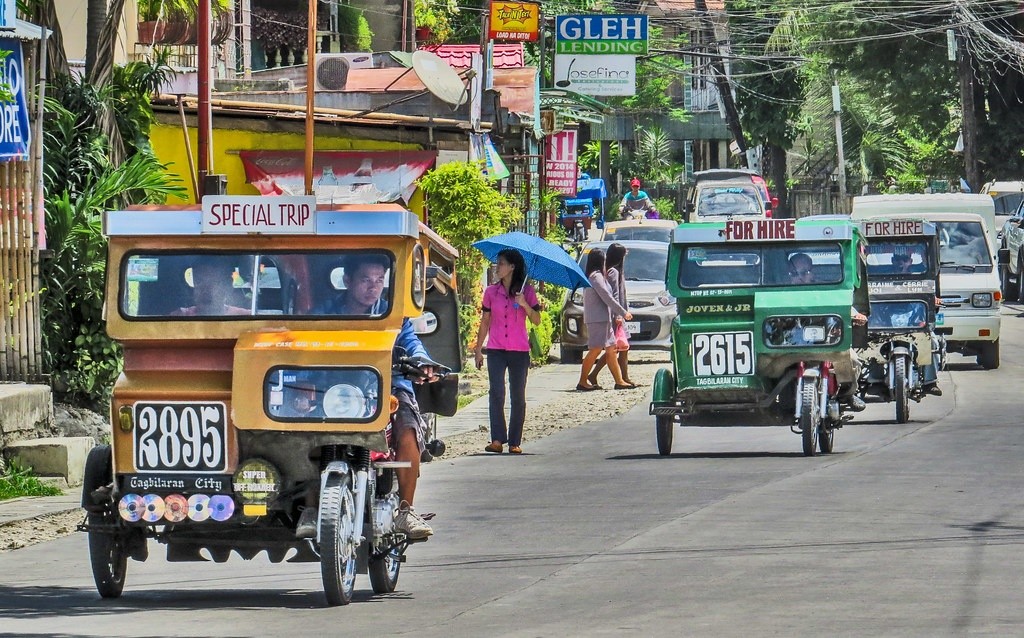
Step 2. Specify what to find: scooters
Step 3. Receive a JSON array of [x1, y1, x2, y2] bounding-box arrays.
[[625, 208, 648, 222]]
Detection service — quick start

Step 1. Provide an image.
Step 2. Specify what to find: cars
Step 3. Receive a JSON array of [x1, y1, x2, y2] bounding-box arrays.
[[600, 219, 678, 243], [560, 241, 673, 363], [997, 196, 1024, 305]]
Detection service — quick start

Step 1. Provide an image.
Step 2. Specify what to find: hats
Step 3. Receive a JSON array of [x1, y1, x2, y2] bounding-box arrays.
[[630, 180, 639, 187]]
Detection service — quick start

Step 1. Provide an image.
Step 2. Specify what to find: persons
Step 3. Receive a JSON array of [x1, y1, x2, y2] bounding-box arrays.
[[171, 260, 250, 316], [776, 254, 870, 411], [474, 249, 541, 453], [576, 242, 638, 391], [294, 251, 439, 539], [874, 248, 946, 395], [618, 179, 656, 216]]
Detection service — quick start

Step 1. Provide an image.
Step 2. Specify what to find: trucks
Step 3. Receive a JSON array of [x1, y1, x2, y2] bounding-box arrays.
[[850, 192, 1002, 371]]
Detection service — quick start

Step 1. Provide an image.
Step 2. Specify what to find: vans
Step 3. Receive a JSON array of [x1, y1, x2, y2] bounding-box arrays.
[[750, 176, 780, 217], [682, 169, 771, 222]]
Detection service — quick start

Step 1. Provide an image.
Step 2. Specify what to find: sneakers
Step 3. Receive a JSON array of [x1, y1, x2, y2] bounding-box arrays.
[[393, 500, 433, 539]]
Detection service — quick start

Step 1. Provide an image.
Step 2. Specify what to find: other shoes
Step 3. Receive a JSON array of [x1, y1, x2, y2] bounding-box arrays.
[[627, 380, 637, 386], [614, 383, 635, 389], [577, 384, 595, 390], [510, 446, 522, 453], [922, 382, 942, 396], [486, 443, 503, 453], [588, 376, 603, 389], [847, 394, 865, 411]]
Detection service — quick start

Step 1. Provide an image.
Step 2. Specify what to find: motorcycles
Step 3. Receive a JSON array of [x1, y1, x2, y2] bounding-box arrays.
[[561, 199, 591, 243], [76, 192, 465, 605], [644, 215, 875, 455], [852, 217, 947, 424]]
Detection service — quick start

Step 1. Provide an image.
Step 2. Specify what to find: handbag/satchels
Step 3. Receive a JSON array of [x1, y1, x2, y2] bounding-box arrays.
[[615, 324, 629, 352]]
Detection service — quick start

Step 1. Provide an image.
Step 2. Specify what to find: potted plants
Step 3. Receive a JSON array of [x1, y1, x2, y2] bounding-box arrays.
[[415, 6, 436, 41], [137, 0, 231, 44]]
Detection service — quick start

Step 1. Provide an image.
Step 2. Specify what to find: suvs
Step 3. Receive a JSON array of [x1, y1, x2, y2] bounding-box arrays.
[[980, 179, 1023, 255]]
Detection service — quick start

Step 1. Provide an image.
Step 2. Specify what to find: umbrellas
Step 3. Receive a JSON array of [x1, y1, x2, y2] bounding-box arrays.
[[469, 231, 593, 309]]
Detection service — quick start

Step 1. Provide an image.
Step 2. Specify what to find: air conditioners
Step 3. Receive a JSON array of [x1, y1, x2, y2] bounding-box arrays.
[[313, 50, 373, 92]]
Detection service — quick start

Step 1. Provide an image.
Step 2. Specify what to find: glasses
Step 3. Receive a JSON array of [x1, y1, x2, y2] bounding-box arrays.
[[894, 255, 910, 262], [789, 268, 812, 275]]
